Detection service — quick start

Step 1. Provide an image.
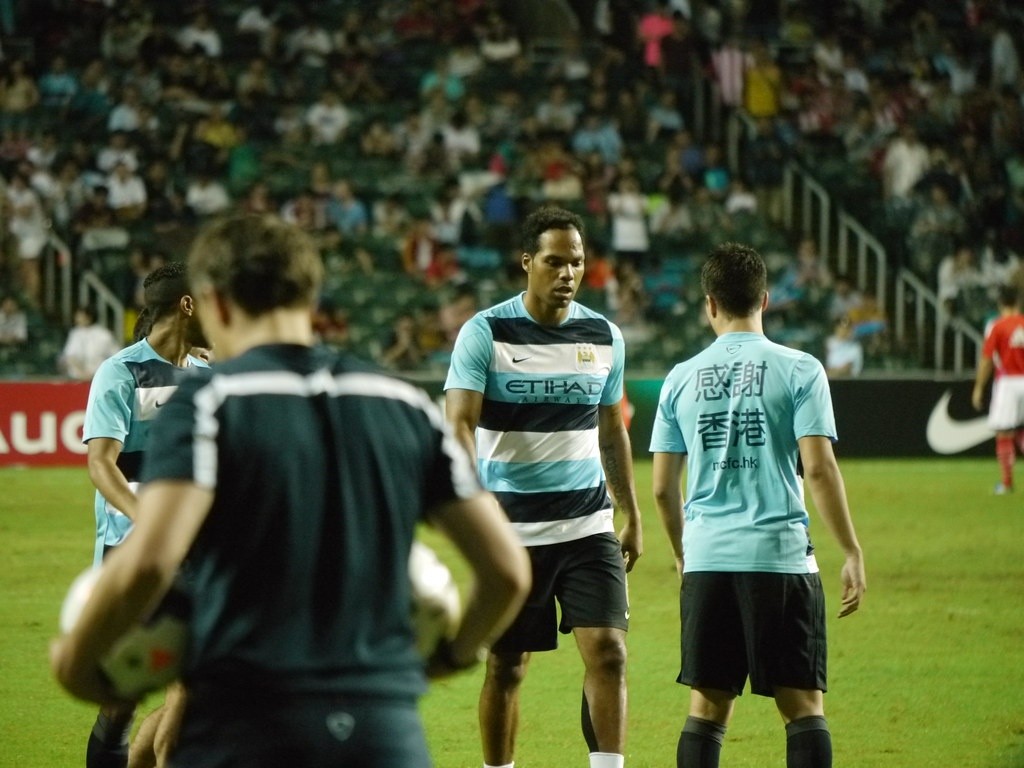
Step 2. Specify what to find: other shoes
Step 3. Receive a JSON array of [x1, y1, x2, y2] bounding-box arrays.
[[994, 484, 1015, 496]]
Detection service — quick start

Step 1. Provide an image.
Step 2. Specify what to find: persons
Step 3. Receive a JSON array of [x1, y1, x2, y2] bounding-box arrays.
[[444, 212, 644, 768], [53, 208, 530, 768], [648, 245, 866, 768], [0, 0, 1024, 378], [972, 288, 1024, 495]]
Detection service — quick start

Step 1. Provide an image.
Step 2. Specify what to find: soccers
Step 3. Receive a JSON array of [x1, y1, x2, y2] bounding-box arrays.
[[61, 561, 194, 701], [405, 541, 464, 663]]
